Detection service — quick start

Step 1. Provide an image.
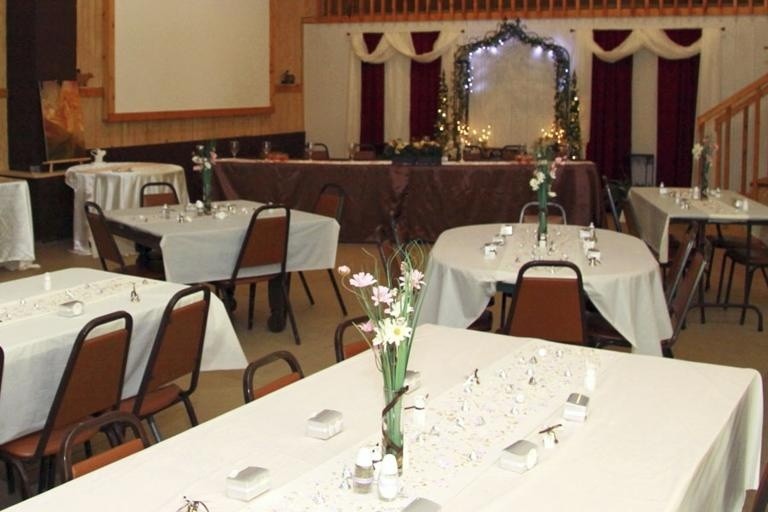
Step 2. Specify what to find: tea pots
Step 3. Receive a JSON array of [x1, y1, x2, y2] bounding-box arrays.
[[90, 148, 106, 165]]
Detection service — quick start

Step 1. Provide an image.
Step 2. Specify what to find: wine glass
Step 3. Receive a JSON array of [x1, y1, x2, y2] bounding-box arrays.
[[347, 143, 357, 161], [304, 141, 315, 163], [261, 141, 272, 162], [228, 140, 241, 160]]
